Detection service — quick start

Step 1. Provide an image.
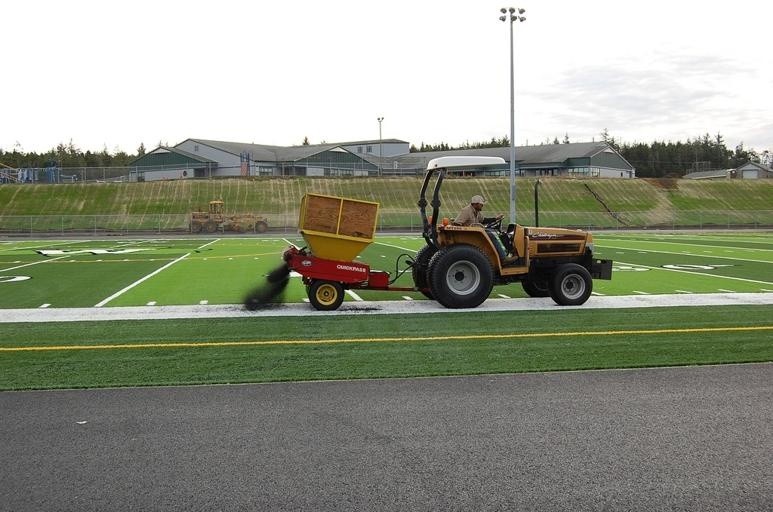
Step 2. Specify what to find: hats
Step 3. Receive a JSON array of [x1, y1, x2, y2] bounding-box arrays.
[[470, 195, 487, 204]]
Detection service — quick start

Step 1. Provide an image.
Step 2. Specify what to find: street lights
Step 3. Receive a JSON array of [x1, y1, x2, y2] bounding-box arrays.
[[377, 116, 387, 178], [499, 6, 526, 222]]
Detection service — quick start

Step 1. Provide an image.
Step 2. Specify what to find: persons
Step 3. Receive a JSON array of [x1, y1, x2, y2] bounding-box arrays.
[[454, 195, 519, 265]]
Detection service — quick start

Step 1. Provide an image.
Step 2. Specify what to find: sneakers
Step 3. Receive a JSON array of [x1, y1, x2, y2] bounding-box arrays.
[[501, 255, 518, 266]]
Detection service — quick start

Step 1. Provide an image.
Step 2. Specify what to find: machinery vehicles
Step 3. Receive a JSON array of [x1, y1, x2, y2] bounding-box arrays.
[[189, 200, 269, 234], [414, 153, 611, 308]]
